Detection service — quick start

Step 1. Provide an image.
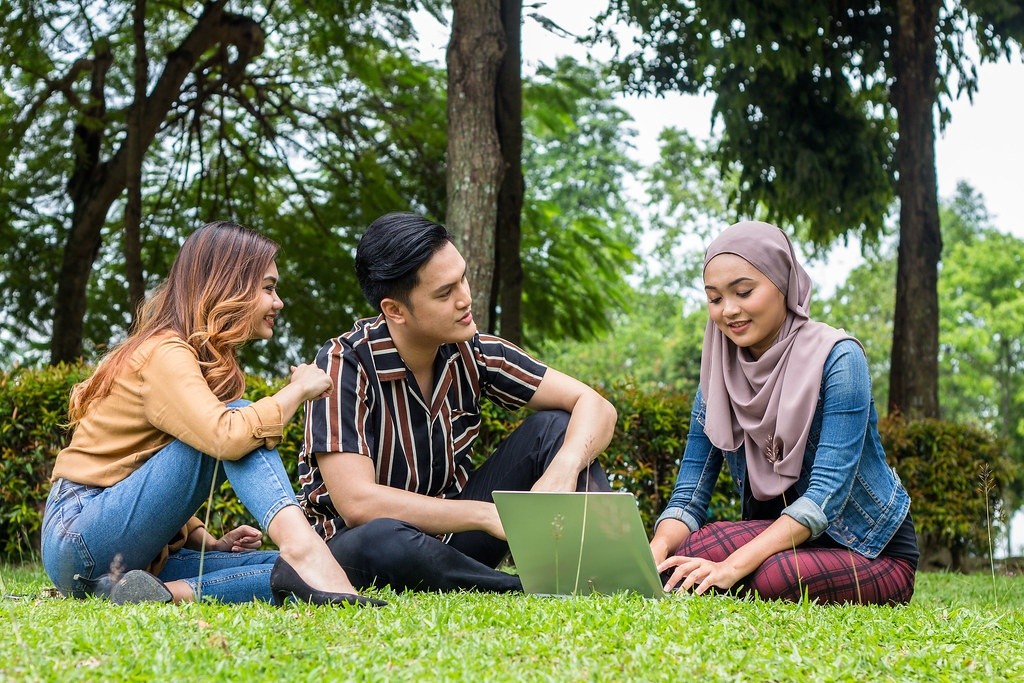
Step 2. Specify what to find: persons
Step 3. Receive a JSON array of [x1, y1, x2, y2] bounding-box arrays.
[[297, 211, 618, 595], [646, 220, 920, 608], [39, 220, 390, 612]]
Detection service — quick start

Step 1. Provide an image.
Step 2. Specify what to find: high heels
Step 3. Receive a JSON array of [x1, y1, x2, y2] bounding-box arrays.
[[72, 568, 176, 605], [270, 558, 390, 609]]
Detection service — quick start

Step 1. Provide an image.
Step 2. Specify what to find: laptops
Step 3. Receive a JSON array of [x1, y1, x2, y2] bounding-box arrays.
[[492, 490, 668, 602]]
